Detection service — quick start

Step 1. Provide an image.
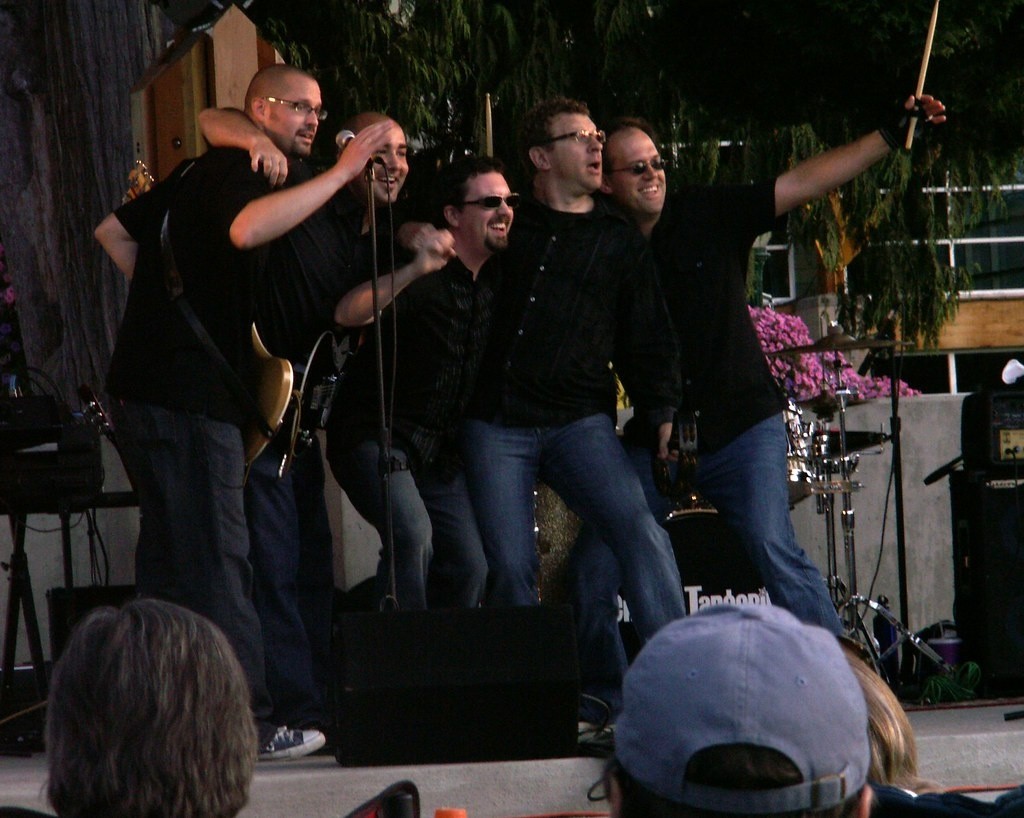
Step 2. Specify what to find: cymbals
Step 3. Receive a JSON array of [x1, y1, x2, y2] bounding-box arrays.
[[765, 333, 913, 358], [815, 429, 893, 455]]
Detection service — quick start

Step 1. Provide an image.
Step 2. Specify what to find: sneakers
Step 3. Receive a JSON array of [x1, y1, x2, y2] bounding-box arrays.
[[258, 726, 325, 767]]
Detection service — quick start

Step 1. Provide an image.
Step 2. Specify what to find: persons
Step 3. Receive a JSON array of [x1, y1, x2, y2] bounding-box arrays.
[[41, 599, 256, 818], [333, 95, 948, 736], [93, 68, 404, 766], [599, 606, 1024, 818]]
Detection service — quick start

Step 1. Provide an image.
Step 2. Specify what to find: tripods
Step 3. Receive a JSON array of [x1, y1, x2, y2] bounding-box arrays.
[[819, 349, 975, 703]]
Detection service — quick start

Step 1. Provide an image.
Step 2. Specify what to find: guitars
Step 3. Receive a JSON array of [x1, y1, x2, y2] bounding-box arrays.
[[122, 158, 296, 463], [75, 380, 116, 445], [290, 332, 348, 456]]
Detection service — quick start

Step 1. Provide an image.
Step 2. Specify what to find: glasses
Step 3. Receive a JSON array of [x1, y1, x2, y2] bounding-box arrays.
[[615, 157, 668, 175], [552, 130, 605, 143], [464, 194, 520, 210], [263, 97, 327, 120]]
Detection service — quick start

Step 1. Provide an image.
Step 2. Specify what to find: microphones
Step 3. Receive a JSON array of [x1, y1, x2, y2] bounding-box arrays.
[[336, 129, 386, 165]]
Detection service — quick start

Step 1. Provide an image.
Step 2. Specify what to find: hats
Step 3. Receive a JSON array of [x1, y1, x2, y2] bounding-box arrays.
[[617, 604, 869, 815]]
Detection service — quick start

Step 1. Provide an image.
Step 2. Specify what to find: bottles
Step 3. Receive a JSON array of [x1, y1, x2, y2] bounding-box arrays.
[[435, 808, 467, 818]]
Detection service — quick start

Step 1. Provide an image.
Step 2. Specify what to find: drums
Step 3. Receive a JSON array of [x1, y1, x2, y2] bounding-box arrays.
[[632, 424, 718, 521], [783, 394, 813, 506]]
